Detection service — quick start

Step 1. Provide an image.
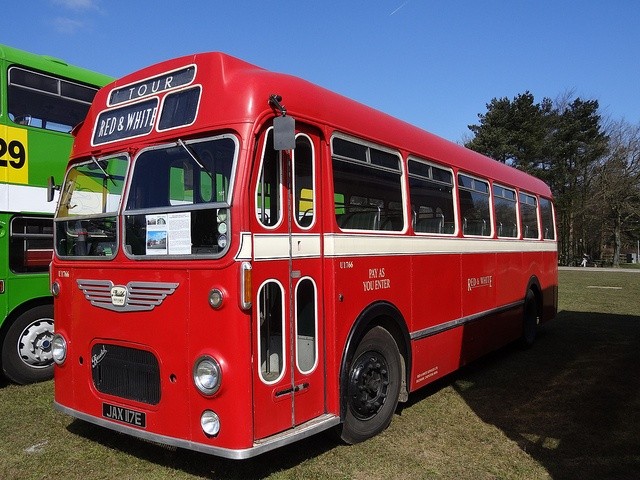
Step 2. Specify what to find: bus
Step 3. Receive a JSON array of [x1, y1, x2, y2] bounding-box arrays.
[[0, 45, 345, 384], [48, 52, 559, 461]]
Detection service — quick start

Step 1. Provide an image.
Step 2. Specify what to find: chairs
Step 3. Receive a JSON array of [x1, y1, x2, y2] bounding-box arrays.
[[575, 253, 612, 267], [601, 252, 634, 267], [301, 208, 551, 239]]
